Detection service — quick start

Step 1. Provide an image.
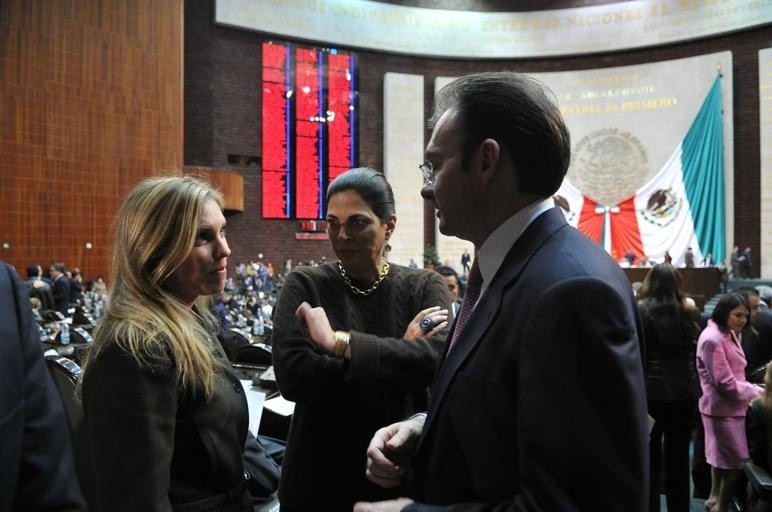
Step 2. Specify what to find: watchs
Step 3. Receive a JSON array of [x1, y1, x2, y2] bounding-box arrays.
[[333, 331, 350, 360]]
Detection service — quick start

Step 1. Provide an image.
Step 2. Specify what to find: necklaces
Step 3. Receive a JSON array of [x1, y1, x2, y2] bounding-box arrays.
[[338, 259, 389, 296]]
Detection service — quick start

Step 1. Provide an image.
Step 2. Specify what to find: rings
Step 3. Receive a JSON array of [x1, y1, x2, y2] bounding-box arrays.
[[419, 317, 434, 333]]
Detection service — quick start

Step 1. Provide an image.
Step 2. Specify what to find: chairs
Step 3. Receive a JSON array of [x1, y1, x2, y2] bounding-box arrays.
[[223, 326, 252, 361], [54, 326, 94, 344], [732, 458, 772, 512], [233, 343, 273, 379], [45, 354, 82, 423]]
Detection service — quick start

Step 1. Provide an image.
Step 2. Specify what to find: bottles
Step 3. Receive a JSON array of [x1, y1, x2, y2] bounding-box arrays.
[[253, 316, 265, 336], [61, 321, 69, 344]]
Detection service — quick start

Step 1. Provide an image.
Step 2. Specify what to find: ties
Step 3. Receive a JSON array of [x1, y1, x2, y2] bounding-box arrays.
[[446, 255, 483, 355]]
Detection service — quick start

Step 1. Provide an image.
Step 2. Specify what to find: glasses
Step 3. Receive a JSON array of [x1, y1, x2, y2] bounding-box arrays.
[[418, 140, 479, 188]]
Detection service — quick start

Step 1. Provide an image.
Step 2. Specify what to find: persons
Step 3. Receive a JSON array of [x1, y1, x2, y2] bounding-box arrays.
[[3, 254, 331, 347], [623, 246, 772, 512], [354, 72, 649, 512], [76, 176, 255, 512], [407, 248, 471, 320], [272, 167, 454, 512], [0, 258, 89, 512]]
